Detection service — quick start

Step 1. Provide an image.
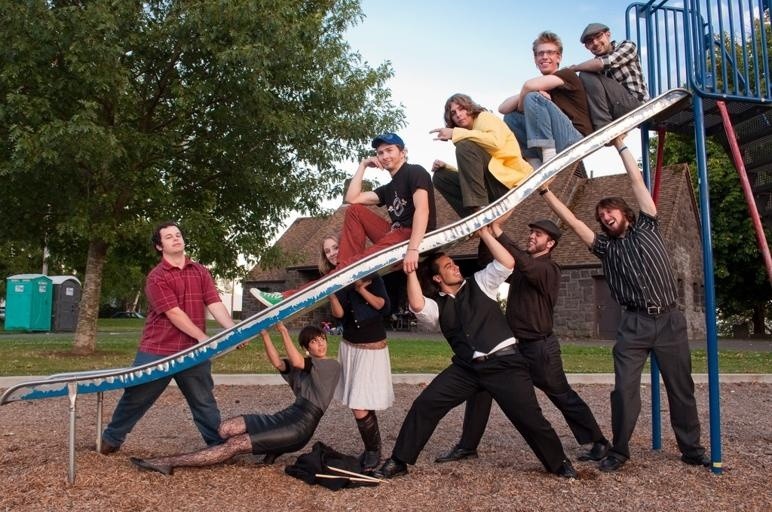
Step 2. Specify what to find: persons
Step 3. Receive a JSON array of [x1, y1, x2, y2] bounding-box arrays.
[[130, 321, 340, 475], [250, 132, 437, 309], [428, 93, 534, 219], [538, 131, 713, 473], [431, 206, 613, 462], [497, 31, 594, 172], [567, 24, 650, 132], [95, 222, 249, 458], [372, 226, 577, 479], [318, 232, 390, 468]]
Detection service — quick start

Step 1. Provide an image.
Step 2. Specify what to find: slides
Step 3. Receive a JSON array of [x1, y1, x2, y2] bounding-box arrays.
[[0, 88, 690, 405]]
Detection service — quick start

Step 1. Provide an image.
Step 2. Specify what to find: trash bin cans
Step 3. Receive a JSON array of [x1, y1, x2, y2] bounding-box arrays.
[[48, 275, 82, 333], [4, 273, 52, 331]]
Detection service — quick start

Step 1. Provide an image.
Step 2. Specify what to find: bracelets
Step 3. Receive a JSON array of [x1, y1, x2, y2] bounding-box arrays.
[[538, 188, 549, 196], [618, 146, 628, 155], [406, 249, 419, 253]]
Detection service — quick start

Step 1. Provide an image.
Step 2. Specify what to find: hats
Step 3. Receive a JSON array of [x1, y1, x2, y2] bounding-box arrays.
[[580, 23, 609, 43], [372, 133, 404, 150], [528, 220, 561, 240]]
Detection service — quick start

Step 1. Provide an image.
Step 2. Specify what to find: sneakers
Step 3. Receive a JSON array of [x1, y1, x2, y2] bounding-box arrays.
[[249, 287, 285, 309]]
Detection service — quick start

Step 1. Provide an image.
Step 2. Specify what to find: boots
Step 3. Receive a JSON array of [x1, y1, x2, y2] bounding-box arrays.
[[356, 409, 381, 475]]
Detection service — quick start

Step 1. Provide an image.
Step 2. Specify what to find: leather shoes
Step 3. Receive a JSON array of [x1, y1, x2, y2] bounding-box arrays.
[[130, 456, 174, 475], [553, 440, 624, 479], [681, 453, 712, 467], [371, 457, 408, 480], [436, 443, 478, 464]]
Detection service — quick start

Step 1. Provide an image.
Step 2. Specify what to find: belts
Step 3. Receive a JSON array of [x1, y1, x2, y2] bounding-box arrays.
[[475, 345, 518, 362], [626, 301, 677, 316]]
